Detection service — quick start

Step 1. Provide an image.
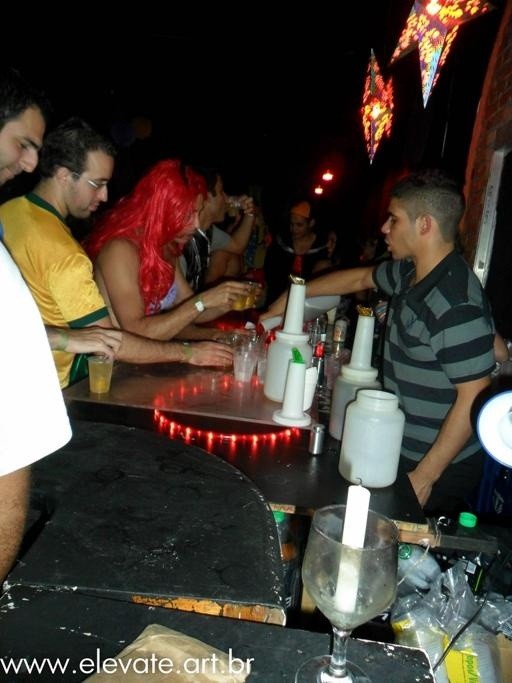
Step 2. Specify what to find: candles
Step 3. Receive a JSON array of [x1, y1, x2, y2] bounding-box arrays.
[[333, 476, 371, 612]]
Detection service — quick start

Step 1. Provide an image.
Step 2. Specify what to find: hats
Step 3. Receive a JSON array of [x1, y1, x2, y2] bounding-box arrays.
[[290, 201, 312, 220]]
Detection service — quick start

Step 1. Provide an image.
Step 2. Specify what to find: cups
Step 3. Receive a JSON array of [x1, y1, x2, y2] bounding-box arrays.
[[225, 196, 239, 217], [88, 356, 113, 394], [391, 593, 512, 683], [232, 344, 258, 383]]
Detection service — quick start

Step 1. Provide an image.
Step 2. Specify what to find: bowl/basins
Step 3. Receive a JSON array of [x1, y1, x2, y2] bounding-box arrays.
[[232, 281, 258, 312]]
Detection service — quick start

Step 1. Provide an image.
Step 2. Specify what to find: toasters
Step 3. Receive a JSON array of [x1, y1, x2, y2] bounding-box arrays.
[[317, 318, 352, 413]]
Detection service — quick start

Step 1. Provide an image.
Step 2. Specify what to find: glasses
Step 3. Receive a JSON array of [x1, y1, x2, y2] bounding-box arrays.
[[70, 171, 105, 193]]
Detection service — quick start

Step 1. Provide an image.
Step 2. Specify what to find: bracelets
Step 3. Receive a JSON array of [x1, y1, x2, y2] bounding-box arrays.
[[182, 341, 192, 363], [53, 325, 68, 353]]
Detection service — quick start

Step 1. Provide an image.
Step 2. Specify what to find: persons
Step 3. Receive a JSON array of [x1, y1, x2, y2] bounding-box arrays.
[[0, 120, 236, 390], [255, 172, 497, 511], [172, 171, 378, 343], [80, 159, 261, 343], [0, 237, 75, 593], [0, 87, 47, 186]]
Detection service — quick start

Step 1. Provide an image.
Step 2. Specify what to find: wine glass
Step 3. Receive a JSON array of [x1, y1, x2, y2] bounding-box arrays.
[[295, 504, 399, 683]]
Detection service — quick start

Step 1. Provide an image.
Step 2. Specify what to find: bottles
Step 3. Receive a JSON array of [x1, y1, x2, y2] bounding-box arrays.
[[329, 315, 382, 442], [398, 544, 442, 590], [263, 283, 313, 403], [338, 389, 406, 488]]
